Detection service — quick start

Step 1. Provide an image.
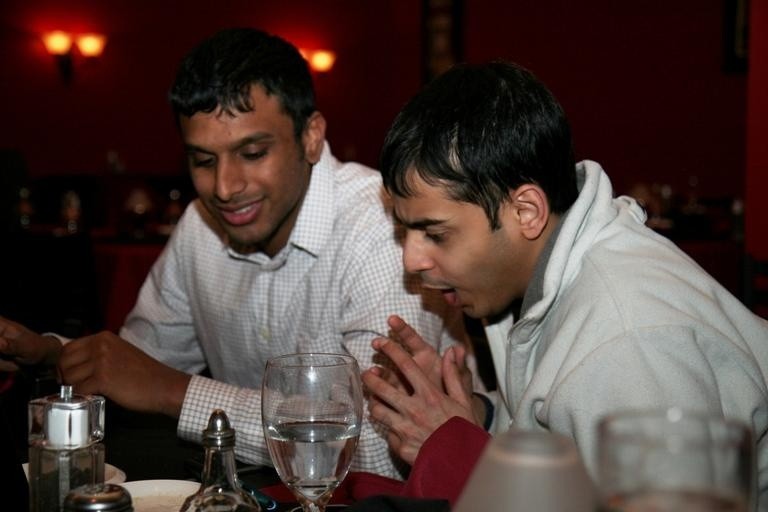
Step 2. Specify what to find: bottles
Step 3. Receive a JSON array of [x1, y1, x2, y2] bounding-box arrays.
[[26, 386, 264, 512]]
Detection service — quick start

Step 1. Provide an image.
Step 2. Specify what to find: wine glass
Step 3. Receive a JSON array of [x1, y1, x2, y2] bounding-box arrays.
[[260, 350, 364, 512]]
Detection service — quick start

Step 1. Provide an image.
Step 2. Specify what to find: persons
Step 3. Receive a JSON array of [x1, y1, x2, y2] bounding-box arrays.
[[1, 27, 494, 482], [359, 60, 767, 511]]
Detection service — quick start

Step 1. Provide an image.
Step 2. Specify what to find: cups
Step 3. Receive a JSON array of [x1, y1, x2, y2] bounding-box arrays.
[[452, 428, 594, 512], [593, 406, 759, 510]]
